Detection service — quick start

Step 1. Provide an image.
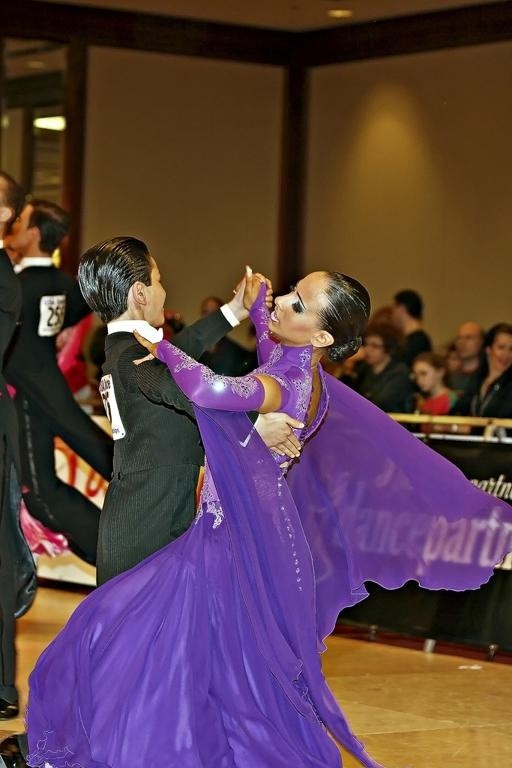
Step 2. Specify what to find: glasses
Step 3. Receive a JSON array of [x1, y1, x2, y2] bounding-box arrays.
[[364, 342, 385, 350]]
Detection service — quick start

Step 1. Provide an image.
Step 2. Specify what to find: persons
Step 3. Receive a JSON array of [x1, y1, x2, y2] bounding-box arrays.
[[76, 236, 306, 593], [28, 264, 372, 768], [1, 165, 109, 719], [58, 317, 108, 416], [165, 295, 258, 377], [324, 289, 511, 435]]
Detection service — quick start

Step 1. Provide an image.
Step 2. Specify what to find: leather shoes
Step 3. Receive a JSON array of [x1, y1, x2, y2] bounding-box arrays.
[[0, 698, 19, 720]]
